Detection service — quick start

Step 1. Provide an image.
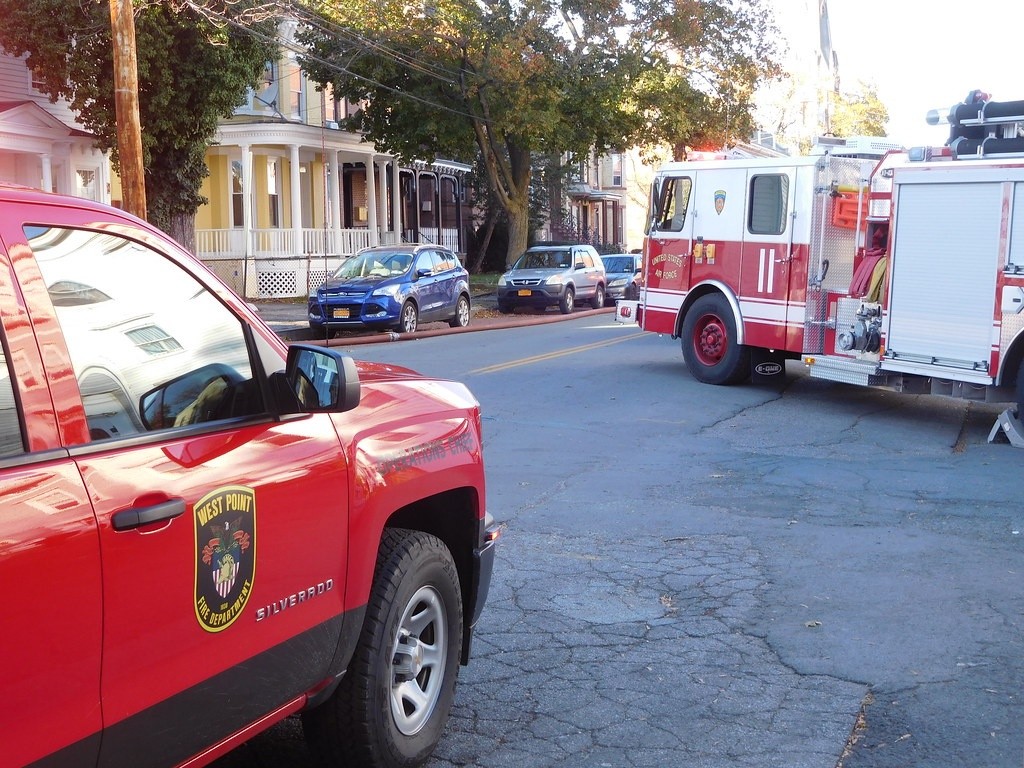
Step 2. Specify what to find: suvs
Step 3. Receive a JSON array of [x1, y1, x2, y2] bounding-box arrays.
[[1, 180, 497, 767], [309, 244, 471, 339]]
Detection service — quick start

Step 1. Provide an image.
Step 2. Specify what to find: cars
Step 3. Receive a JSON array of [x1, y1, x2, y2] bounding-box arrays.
[[497, 245, 607, 314], [599, 254, 642, 303]]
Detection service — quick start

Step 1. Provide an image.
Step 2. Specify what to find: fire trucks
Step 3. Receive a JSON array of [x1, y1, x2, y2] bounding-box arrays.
[[614, 89, 1024, 449]]
